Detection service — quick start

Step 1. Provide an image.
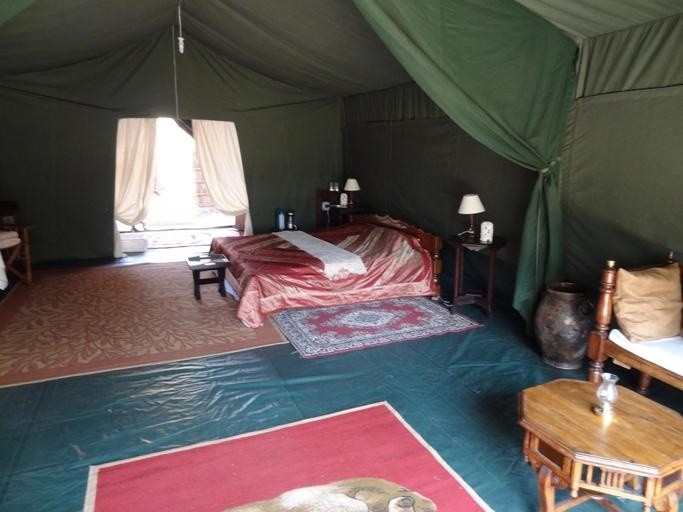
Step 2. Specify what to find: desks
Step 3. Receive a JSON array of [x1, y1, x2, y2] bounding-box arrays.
[[515, 376, 683, 512], [446, 234, 507, 321], [322, 204, 360, 230]]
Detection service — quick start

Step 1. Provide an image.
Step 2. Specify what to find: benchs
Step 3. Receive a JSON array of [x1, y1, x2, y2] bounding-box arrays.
[[585, 248, 682, 396]]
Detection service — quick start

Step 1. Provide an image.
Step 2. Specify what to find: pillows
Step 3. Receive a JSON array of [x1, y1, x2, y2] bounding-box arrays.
[[609, 261, 681, 343]]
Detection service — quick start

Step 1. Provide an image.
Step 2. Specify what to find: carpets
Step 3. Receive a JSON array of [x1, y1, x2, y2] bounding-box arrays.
[[0, 259, 290, 390], [270, 292, 485, 361], [80, 399, 496, 512]]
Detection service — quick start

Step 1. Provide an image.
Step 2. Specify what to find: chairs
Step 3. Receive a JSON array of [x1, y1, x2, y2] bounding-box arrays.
[[0, 200, 33, 288]]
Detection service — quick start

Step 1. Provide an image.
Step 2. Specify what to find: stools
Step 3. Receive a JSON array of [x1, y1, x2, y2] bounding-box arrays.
[[185, 253, 229, 300]]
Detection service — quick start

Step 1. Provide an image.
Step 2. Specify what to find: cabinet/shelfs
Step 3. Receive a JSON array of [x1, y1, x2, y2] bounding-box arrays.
[[314, 186, 342, 229]]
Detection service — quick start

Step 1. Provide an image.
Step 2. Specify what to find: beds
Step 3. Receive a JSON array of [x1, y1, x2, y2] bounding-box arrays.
[[208, 212, 442, 330]]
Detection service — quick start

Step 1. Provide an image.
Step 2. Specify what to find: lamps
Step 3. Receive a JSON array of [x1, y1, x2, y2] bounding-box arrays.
[[343, 177, 360, 206], [456, 192, 485, 237], [176, 3, 186, 54]]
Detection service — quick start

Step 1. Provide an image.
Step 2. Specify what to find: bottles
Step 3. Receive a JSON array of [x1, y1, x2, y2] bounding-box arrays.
[[287, 208, 295, 230], [592, 372, 619, 415], [277, 211, 284, 230]]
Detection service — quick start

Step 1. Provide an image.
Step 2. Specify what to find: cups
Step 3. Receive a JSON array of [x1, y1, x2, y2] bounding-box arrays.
[[328, 181, 339, 192]]
